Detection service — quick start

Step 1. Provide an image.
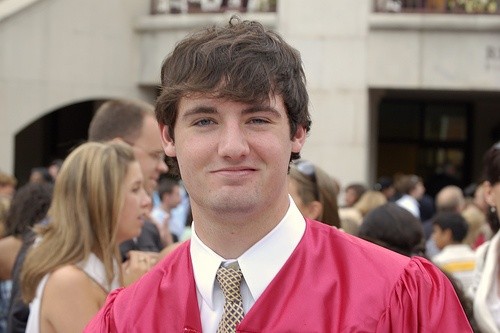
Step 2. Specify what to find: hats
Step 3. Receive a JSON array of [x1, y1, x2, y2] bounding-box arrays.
[[356, 202, 423, 258]]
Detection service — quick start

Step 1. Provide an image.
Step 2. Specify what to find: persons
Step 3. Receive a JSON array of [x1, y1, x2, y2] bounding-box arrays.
[[289, 144, 500, 333], [0, 99, 193, 333], [21, 137, 157, 333], [80, 15, 474, 333]]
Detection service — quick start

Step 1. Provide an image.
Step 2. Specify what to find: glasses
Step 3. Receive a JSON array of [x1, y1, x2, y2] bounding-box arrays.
[[292, 159, 320, 202]]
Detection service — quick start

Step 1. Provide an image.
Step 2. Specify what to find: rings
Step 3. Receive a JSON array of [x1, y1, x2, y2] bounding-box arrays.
[[138, 256, 148, 263]]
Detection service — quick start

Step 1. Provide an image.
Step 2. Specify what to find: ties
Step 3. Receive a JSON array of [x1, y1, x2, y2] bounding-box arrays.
[[216, 261, 245, 333]]
